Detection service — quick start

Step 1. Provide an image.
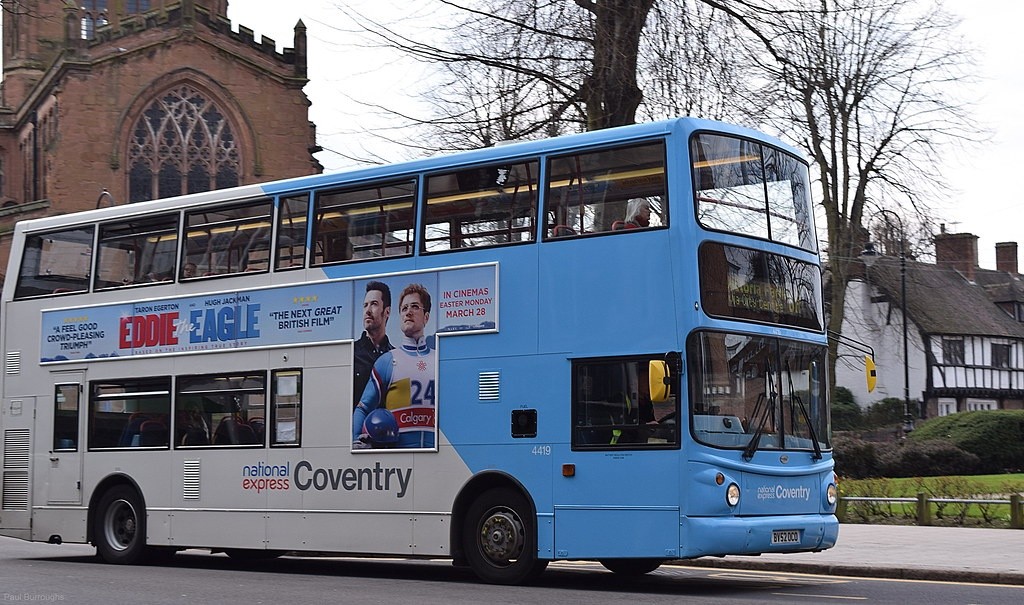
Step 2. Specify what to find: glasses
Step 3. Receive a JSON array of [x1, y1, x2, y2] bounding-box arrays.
[[399, 303, 425, 314]]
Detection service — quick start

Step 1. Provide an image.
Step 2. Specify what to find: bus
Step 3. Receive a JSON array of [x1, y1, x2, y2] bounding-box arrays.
[[0, 116, 878, 577]]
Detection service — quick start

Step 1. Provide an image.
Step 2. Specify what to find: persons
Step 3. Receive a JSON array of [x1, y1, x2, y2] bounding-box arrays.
[[183, 263, 197, 278], [326, 238, 354, 261], [352, 280, 436, 449], [622, 198, 651, 229], [616, 372, 660, 424]]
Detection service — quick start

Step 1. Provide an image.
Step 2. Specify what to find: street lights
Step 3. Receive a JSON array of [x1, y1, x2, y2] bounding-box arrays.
[[858, 209, 916, 444]]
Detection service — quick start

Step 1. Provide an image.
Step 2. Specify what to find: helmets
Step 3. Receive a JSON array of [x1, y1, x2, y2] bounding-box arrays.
[[362, 408, 400, 442]]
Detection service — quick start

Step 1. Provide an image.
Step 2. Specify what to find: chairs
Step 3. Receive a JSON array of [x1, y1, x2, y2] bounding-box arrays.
[[117, 409, 275, 446], [553, 218, 629, 237]]
